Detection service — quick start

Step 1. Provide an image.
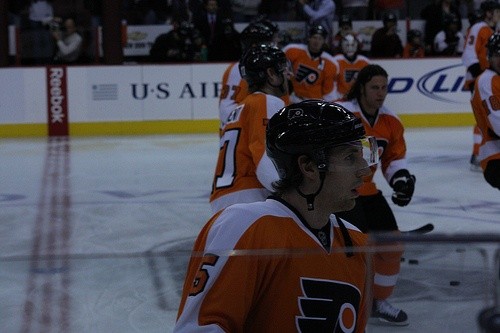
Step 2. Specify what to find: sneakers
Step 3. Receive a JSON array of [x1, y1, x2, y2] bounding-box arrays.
[[370, 297, 409, 326], [470, 154, 483, 172]]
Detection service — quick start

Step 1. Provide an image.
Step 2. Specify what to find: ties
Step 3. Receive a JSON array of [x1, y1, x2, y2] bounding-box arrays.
[[210, 15, 213, 23]]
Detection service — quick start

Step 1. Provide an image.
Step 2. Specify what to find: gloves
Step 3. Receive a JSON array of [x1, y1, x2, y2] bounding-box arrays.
[[484, 159, 500, 189], [389, 169, 415, 206]]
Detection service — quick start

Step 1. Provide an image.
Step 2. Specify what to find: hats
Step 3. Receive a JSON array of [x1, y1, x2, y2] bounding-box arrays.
[[384, 13, 396, 19], [310, 25, 328, 35], [241, 21, 279, 54]]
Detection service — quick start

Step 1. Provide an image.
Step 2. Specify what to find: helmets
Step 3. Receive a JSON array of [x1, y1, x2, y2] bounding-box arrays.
[[266, 100, 365, 179], [244, 44, 287, 72], [480, 0, 500, 10], [485, 32, 500, 49]]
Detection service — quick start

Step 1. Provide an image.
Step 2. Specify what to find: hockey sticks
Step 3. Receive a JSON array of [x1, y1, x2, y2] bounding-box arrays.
[[401, 248, 454, 268], [399, 222, 434, 233]]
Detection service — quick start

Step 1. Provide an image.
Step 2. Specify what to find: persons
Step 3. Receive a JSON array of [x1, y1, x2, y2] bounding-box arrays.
[[0, 0, 500, 333]]
